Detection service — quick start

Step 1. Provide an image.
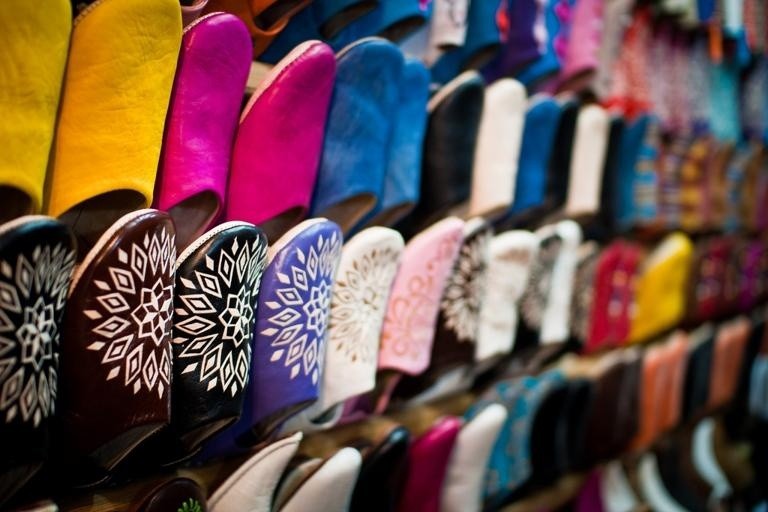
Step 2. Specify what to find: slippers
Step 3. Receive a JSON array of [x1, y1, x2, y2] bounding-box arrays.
[[1, 0, 768, 254], [3, 217, 768, 512]]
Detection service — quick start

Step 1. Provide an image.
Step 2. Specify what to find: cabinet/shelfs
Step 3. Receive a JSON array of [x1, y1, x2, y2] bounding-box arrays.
[[1, 55, 766, 512]]
